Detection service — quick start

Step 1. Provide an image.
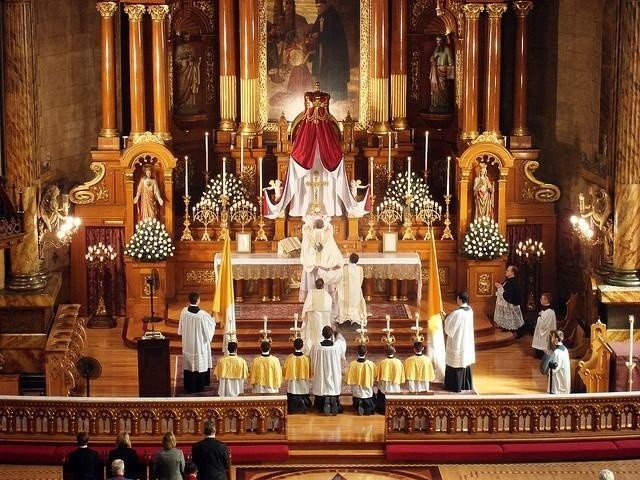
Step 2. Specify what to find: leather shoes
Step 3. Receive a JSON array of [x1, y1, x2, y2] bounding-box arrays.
[[331, 397, 338, 416], [358, 400, 364, 415], [324, 397, 330, 416], [364, 400, 371, 415]]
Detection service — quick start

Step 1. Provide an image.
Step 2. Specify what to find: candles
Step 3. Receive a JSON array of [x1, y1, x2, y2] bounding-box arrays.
[[361, 316, 364, 331], [376, 199, 403, 216], [424, 130, 429, 173], [415, 198, 442, 217], [263, 316, 267, 331], [370, 156, 374, 198], [184, 154, 190, 198], [240, 132, 245, 179], [192, 200, 220, 219], [628, 313, 634, 367], [229, 200, 257, 218], [386, 315, 390, 329], [222, 156, 227, 195], [415, 311, 419, 328], [388, 131, 392, 172], [446, 156, 451, 197], [407, 156, 411, 196], [228, 317, 232, 331], [293, 312, 298, 329], [204, 131, 209, 175], [259, 156, 263, 200]]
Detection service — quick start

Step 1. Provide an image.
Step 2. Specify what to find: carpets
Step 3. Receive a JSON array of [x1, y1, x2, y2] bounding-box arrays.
[[236, 301, 410, 319]]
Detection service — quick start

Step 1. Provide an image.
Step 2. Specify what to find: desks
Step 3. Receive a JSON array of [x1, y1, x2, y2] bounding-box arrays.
[[214, 251, 422, 303]]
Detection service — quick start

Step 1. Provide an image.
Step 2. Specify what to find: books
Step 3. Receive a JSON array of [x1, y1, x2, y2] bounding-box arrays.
[[280, 236, 300, 252]]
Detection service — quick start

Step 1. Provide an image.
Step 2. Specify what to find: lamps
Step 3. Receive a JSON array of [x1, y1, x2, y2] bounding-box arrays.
[[570, 185, 611, 244], [41, 186, 81, 247]]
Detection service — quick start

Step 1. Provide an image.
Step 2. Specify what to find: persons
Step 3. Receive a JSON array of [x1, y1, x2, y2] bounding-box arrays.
[[134, 168, 163, 220], [403, 342, 435, 395], [249, 342, 282, 393], [301, 278, 332, 357], [108, 460, 131, 480], [212, 343, 248, 396], [39, 184, 66, 241], [177, 292, 218, 393], [191, 422, 231, 480], [308, 326, 343, 417], [282, 338, 314, 414], [315, 253, 368, 329], [106, 431, 143, 480], [299, 217, 343, 304], [174, 31, 206, 116], [474, 162, 493, 218], [494, 266, 526, 337], [64, 431, 104, 479], [344, 345, 378, 416], [440, 290, 474, 393], [376, 346, 406, 415], [149, 431, 185, 479], [540, 330, 571, 394], [577, 184, 613, 232], [599, 468, 616, 479], [428, 35, 454, 114], [532, 293, 555, 358], [182, 462, 198, 480]]
